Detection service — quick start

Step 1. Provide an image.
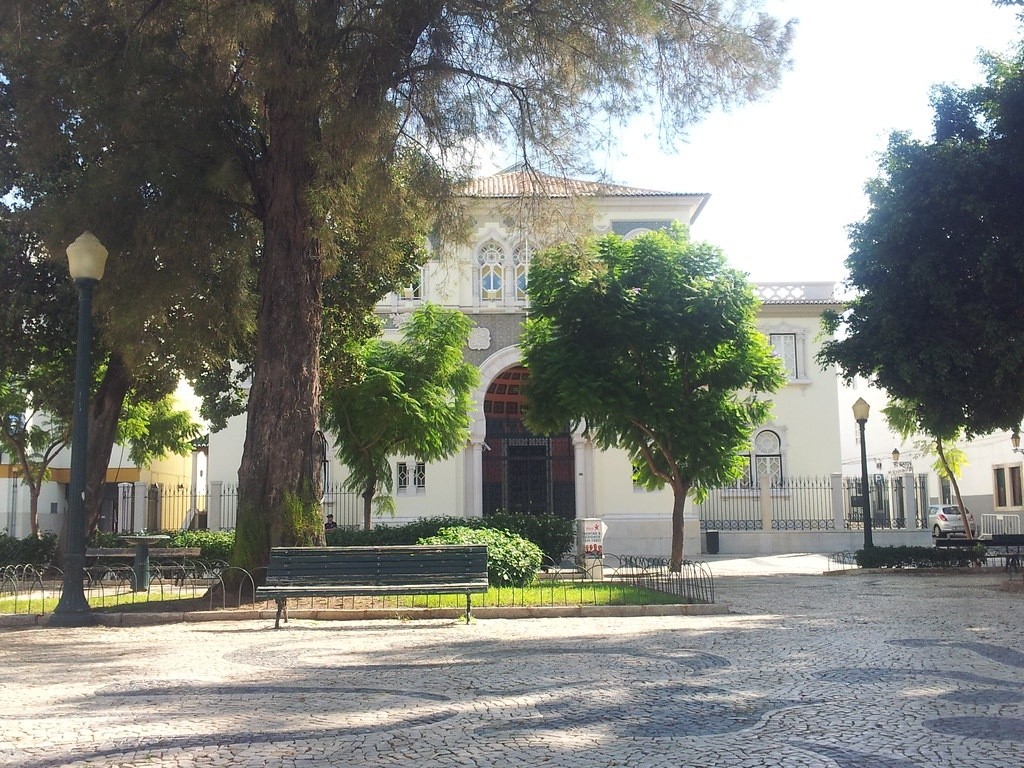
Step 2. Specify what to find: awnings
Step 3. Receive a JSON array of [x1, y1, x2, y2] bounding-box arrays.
[[0, 462, 140, 484]]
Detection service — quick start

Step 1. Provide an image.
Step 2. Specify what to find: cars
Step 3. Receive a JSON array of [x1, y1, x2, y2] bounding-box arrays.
[[927, 505, 976, 538]]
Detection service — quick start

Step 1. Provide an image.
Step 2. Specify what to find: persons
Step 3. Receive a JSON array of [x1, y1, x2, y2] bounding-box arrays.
[[323, 513, 339, 529]]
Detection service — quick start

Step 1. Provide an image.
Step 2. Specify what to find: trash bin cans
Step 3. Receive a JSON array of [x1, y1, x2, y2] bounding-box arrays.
[[706, 530, 719, 554]]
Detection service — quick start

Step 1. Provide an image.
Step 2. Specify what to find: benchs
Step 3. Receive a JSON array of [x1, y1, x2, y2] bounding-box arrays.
[[85, 548, 201, 586], [935, 534, 1024, 570], [255, 544, 489, 625]]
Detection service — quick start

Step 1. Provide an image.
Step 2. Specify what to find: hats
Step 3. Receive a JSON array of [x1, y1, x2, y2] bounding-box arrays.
[[326, 514, 333, 517]]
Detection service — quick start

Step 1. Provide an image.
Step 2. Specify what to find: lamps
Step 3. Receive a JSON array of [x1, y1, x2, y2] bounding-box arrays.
[[891, 448, 912, 471], [1011, 433, 1024, 455]]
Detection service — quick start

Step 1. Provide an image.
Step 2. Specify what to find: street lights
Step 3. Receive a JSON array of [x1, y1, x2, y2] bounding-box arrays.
[[852, 397, 875, 547], [36, 231, 109, 629]]
